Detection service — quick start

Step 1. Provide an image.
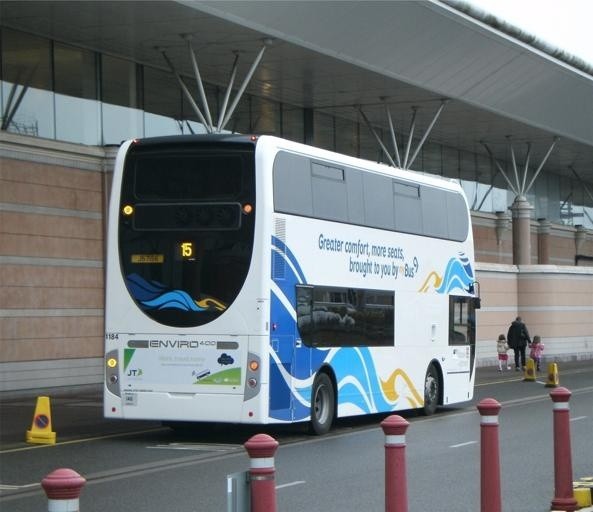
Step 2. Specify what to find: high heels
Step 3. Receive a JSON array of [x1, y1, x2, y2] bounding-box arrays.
[[544, 361, 560, 388], [523, 357, 537, 382], [26, 395, 57, 446]]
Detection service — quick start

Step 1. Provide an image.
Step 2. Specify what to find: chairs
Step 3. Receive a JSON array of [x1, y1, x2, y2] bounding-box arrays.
[[498, 366, 526, 371]]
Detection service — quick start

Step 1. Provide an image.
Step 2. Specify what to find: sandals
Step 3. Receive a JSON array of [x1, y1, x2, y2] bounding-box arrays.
[[103, 133, 481, 435]]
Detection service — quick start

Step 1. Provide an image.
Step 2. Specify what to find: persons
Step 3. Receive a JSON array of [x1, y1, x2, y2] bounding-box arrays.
[[496, 334, 512, 371], [529, 336, 544, 371], [507, 316, 531, 371]]
